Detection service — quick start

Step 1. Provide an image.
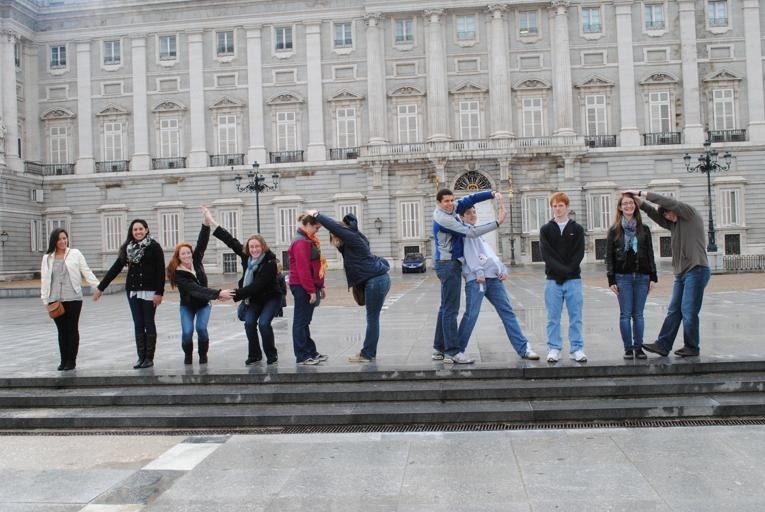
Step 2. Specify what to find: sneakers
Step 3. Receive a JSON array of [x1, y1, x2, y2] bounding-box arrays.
[[296, 358, 319, 365], [432, 349, 444, 360], [444, 352, 475, 364], [520, 342, 540, 360], [185, 357, 192, 364], [624, 351, 633, 359], [636, 350, 647, 359], [315, 354, 328, 361], [348, 355, 370, 363], [568, 351, 587, 362], [547, 349, 560, 362], [246, 356, 262, 365], [267, 349, 277, 364], [200, 355, 207, 364]]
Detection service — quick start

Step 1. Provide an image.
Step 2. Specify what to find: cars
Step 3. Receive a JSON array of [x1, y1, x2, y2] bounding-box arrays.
[[401, 253, 426, 273]]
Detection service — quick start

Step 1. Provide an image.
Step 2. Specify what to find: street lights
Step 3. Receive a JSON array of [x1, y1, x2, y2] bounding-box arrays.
[[235, 161, 280, 234], [683, 140, 732, 251]]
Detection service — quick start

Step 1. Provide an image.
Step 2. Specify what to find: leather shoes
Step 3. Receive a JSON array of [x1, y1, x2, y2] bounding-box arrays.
[[675, 349, 699, 356], [642, 344, 668, 357], [138, 359, 153, 368], [64, 364, 75, 370], [133, 360, 144, 368], [58, 364, 65, 370]]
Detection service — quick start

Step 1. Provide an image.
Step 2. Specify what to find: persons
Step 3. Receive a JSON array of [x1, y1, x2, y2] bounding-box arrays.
[[203, 202, 287, 365], [287, 216, 328, 366], [539, 194, 589, 363], [310, 210, 391, 362], [430, 190, 506, 363], [95, 220, 166, 368], [621, 190, 710, 358], [605, 195, 657, 361], [164, 211, 236, 365], [40, 230, 99, 369], [454, 203, 540, 360]]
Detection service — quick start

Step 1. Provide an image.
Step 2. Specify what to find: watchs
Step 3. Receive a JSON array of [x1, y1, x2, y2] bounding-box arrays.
[[637, 190, 642, 196]]
